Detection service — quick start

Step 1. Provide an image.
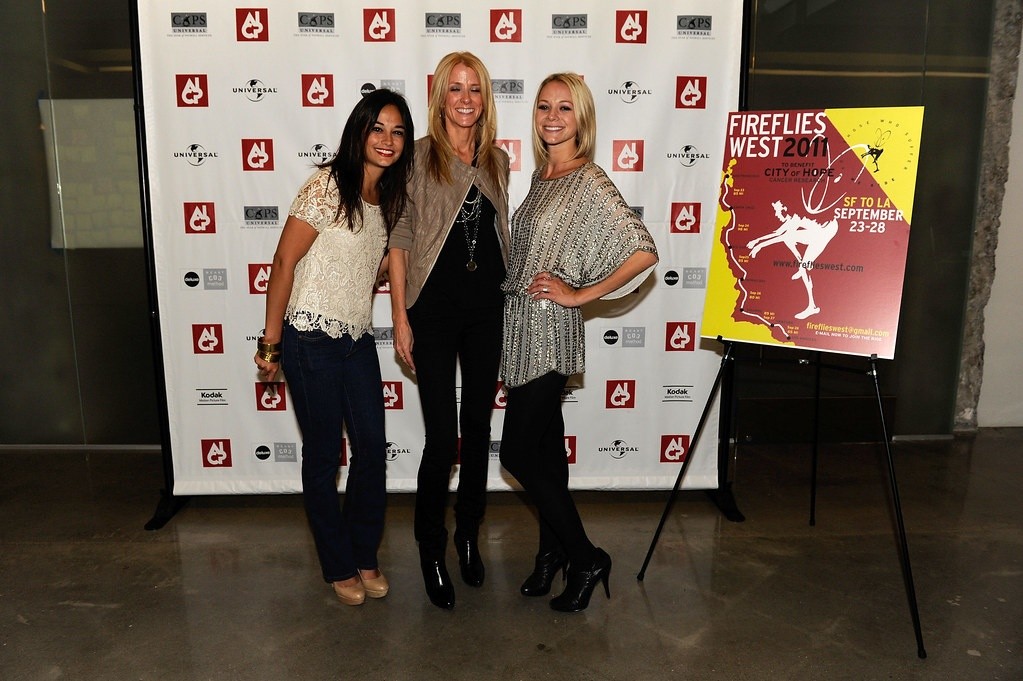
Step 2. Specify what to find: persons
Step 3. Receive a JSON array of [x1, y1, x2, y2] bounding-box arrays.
[[499, 73, 659, 612], [390, 52, 510, 609], [254, 88, 415, 605]]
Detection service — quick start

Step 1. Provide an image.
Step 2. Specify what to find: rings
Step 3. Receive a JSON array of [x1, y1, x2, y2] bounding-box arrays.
[[399, 355, 406, 360], [543, 287, 548, 293], [549, 274, 554, 279]]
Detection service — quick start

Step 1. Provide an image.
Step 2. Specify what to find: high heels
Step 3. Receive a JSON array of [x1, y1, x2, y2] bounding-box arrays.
[[550, 546, 611, 612], [520, 550, 570, 596]]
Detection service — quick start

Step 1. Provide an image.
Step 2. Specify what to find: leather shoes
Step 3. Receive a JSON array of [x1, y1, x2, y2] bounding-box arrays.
[[420, 553, 455, 609], [454, 531, 485, 587]]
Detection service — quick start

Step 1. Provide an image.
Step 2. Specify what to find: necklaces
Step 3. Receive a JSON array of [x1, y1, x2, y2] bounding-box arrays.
[[455, 190, 481, 271]]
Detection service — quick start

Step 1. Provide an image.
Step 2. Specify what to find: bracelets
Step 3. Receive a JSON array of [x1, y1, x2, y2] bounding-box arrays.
[[258, 337, 281, 351], [257, 350, 280, 363]]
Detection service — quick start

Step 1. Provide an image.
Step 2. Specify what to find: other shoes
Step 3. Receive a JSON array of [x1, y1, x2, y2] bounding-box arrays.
[[333, 582, 366, 606], [356, 569, 389, 598]]
